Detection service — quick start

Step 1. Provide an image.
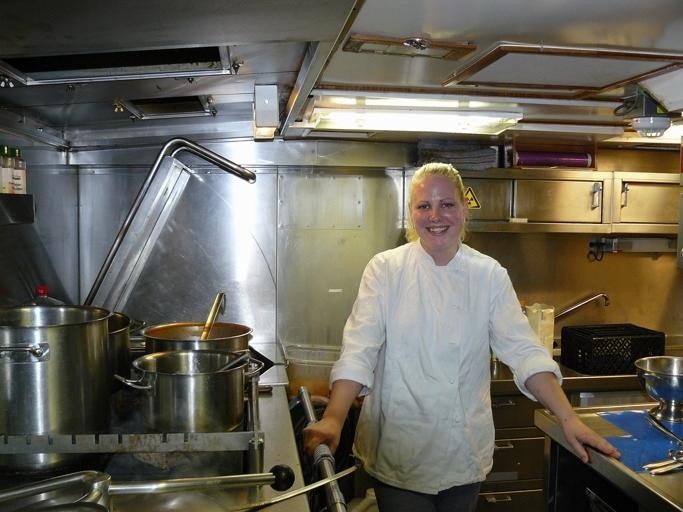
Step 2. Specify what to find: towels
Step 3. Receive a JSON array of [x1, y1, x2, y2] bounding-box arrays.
[[304, 421, 335, 491]]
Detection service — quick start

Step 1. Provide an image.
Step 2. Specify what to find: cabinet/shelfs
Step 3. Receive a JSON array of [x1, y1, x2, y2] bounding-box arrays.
[[403, 166, 680, 234], [478, 395, 546, 512]]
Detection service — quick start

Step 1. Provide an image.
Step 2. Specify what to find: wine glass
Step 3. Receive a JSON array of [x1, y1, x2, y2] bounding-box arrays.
[[634, 354, 683, 424]]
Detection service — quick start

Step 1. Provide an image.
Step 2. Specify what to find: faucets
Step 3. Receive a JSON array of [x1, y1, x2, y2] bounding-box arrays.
[[551, 292, 612, 322]]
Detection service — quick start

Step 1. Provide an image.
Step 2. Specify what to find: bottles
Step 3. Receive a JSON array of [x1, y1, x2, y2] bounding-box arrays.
[[0, 143, 28, 194]]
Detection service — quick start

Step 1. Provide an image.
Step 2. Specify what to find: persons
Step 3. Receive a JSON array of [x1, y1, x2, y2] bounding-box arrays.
[[301, 160, 621, 512]]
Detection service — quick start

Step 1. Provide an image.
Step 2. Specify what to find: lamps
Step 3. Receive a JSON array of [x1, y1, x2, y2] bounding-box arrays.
[[303, 89, 523, 135], [253, 84, 278, 142]]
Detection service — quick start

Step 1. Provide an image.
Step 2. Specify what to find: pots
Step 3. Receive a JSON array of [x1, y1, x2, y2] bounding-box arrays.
[[1, 304, 254, 431]]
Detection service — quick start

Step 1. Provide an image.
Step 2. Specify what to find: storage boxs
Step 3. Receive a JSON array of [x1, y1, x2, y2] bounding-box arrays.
[[286, 344, 341, 401]]
